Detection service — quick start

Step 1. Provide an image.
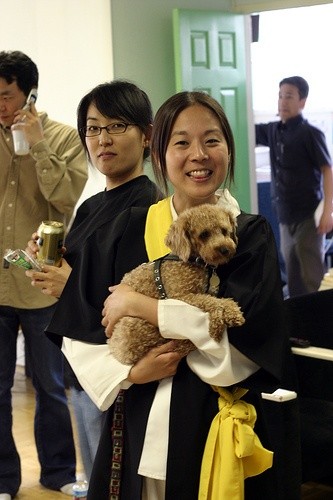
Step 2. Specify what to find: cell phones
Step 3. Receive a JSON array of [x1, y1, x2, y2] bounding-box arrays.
[[23, 89, 38, 111]]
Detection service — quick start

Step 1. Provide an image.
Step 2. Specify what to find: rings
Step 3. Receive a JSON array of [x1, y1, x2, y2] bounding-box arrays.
[[20, 115, 27, 121]]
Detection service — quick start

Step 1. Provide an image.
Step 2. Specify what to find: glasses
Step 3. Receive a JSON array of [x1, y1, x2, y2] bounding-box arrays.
[[79, 121, 136, 137]]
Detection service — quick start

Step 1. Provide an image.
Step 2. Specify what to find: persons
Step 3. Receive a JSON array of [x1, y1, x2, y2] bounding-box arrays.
[[25, 80, 167, 500], [254, 75, 333, 297], [44, 91, 298, 500], [0, 50, 89, 500]]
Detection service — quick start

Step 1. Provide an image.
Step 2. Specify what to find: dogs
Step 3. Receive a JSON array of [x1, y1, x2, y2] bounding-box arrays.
[[107, 203, 246, 365]]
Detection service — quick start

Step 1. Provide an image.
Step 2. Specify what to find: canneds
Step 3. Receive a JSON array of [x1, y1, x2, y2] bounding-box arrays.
[[35, 220, 65, 267]]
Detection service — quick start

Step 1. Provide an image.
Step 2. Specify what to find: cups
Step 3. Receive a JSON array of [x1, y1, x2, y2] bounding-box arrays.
[[12, 123, 30, 156]]
